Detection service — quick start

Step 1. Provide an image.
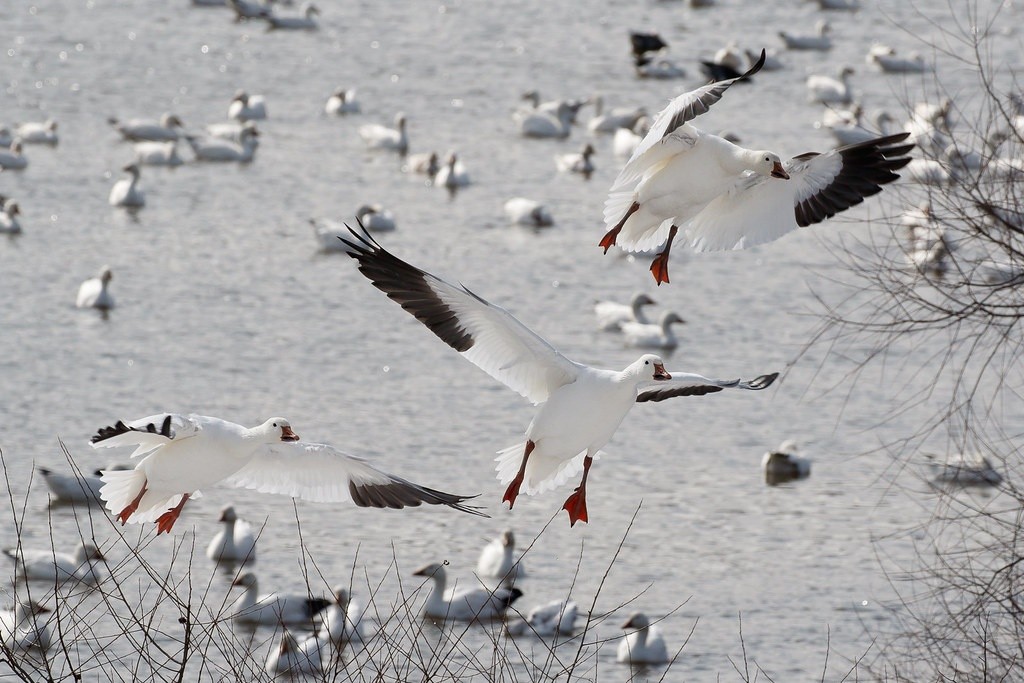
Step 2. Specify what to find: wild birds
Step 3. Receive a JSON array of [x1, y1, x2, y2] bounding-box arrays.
[[617, 311, 687, 351], [231, 572, 334, 625], [87, 413, 492, 537], [407, 151, 440, 176], [359, 204, 395, 231], [777, 1, 1024, 287], [206, 506, 257, 561], [356, 111, 409, 152], [761, 440, 811, 488], [504, 196, 554, 226], [108, 163, 145, 209], [500, 598, 579, 637], [0, 199, 23, 233], [265, 630, 321, 673], [926, 451, 1003, 486], [477, 529, 525, 579], [105, 90, 268, 167], [514, 31, 783, 173], [597, 48, 917, 287], [225, 0, 320, 29], [434, 151, 471, 189], [336, 214, 781, 529], [75, 266, 115, 309], [324, 85, 361, 116], [0, 119, 59, 171], [37, 464, 136, 504], [593, 293, 659, 333], [308, 204, 378, 252], [411, 562, 524, 621], [2, 541, 107, 582], [0, 600, 52, 650], [615, 611, 668, 663], [319, 585, 365, 643]]
